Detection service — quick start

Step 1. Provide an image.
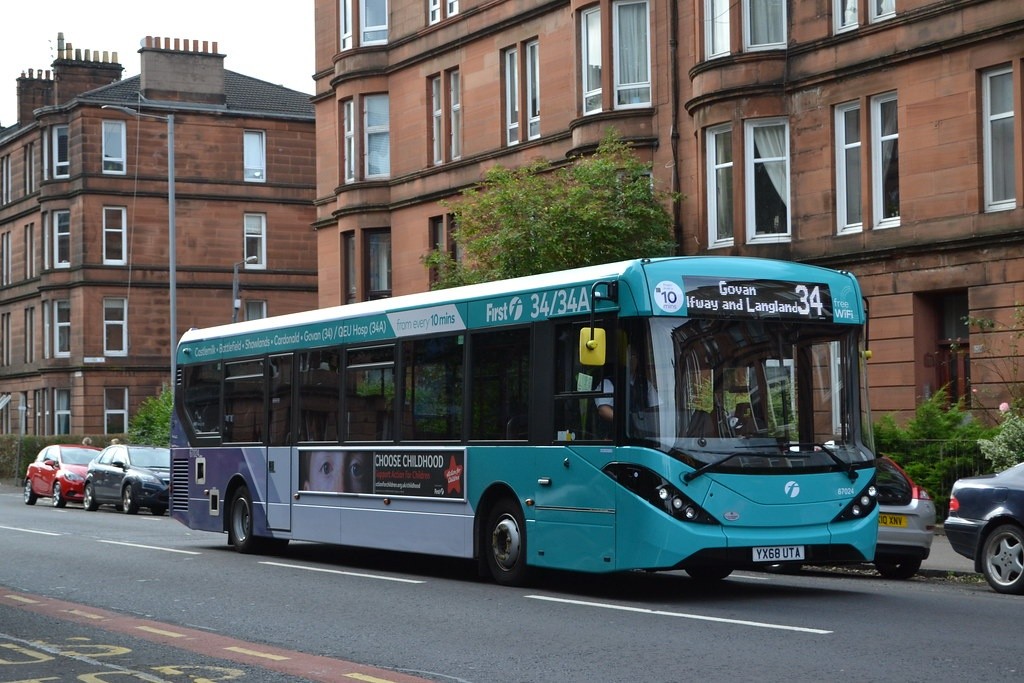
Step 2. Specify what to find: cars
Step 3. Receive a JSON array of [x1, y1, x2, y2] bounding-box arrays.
[[943, 462, 1024, 594], [758, 440, 937, 580], [24, 444, 104, 508], [83, 443, 171, 516]]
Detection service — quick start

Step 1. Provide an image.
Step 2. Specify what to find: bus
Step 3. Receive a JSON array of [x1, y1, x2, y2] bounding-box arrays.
[[169, 255, 880, 587]]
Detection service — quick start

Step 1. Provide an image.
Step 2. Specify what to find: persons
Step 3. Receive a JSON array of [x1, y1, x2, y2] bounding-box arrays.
[[302, 452, 371, 494], [593, 345, 657, 442]]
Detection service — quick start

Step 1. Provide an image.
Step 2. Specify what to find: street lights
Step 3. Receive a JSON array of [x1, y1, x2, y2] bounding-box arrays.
[[103, 105, 177, 403], [232, 256, 258, 323]]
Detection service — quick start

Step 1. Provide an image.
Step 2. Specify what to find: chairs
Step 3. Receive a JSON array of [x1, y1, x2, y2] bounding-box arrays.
[[506, 414, 529, 440]]
